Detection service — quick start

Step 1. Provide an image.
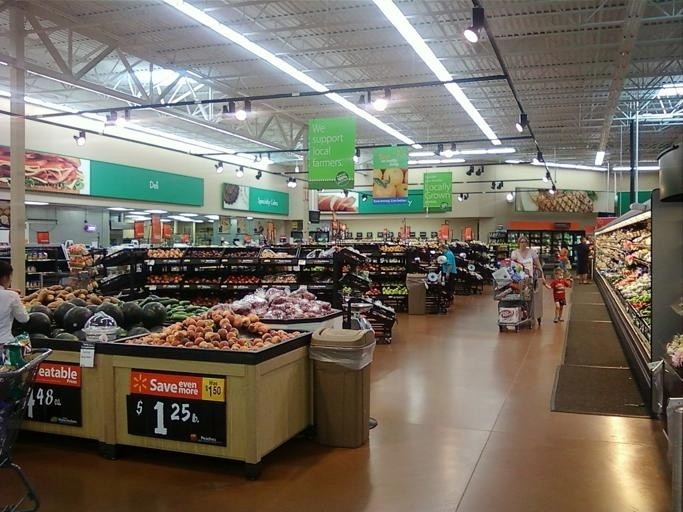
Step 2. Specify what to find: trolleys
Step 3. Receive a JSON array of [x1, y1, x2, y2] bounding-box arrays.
[[0, 346, 54, 511], [497, 278, 544, 331]]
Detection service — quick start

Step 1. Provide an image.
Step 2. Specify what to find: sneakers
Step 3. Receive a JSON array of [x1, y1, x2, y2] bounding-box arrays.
[[559, 317, 564, 321], [554, 317, 558, 322]]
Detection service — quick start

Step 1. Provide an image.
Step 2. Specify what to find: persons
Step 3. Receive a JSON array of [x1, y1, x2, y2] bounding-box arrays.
[[576, 237, 593, 285], [438, 244, 457, 306], [511, 235, 546, 287], [1, 261, 30, 347], [545, 268, 571, 321]]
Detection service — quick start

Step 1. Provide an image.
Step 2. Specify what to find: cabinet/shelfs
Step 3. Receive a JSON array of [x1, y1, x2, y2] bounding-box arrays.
[[0, 243, 72, 296], [148, 247, 335, 310], [335, 247, 395, 342], [264, 306, 344, 355], [508, 228, 593, 271], [369, 244, 412, 311], [98, 248, 145, 303], [494, 276, 537, 333], [0, 300, 106, 460], [105, 325, 314, 480], [594, 199, 681, 415]]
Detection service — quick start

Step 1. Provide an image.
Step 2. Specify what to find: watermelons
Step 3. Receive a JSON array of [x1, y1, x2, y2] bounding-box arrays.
[[69, 298, 88, 307], [24, 311, 52, 334], [120, 302, 142, 324], [63, 306, 93, 332], [55, 332, 79, 340], [53, 301, 76, 324], [141, 302, 167, 327], [52, 328, 71, 338], [31, 305, 53, 322], [94, 301, 125, 328]]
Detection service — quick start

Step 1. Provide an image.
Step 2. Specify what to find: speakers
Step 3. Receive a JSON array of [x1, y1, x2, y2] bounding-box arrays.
[[309, 211, 320, 223]]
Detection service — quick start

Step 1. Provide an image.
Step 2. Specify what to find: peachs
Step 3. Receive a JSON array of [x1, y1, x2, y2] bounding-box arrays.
[[125, 332, 163, 346], [163, 309, 301, 352]]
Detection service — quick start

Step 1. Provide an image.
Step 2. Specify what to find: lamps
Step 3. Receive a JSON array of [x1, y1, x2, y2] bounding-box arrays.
[[228, 102, 236, 116], [76, 131, 86, 146], [457, 194, 463, 202], [361, 194, 367, 202], [464, 166, 474, 176], [532, 152, 543, 165], [373, 88, 392, 111], [549, 185, 557, 195], [292, 179, 296, 188], [287, 178, 292, 188], [353, 149, 361, 162], [238, 167, 243, 178], [464, 8, 485, 43], [496, 181, 504, 192], [542, 172, 550, 182], [254, 171, 262, 180], [106, 111, 118, 132], [217, 162, 224, 173], [515, 113, 527, 133], [123, 110, 132, 128], [447, 142, 458, 155], [490, 181, 496, 190], [475, 166, 486, 176], [433, 143, 443, 156]]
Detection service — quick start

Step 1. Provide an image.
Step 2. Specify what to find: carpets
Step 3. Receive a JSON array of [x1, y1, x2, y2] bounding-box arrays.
[[571, 292, 604, 303], [564, 321, 630, 367], [550, 366, 652, 421], [573, 285, 599, 293], [571, 303, 611, 321]]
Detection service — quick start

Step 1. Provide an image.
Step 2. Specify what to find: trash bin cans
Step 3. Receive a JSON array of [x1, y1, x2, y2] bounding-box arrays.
[[310, 326, 376, 449], [406, 273, 428, 315]]
